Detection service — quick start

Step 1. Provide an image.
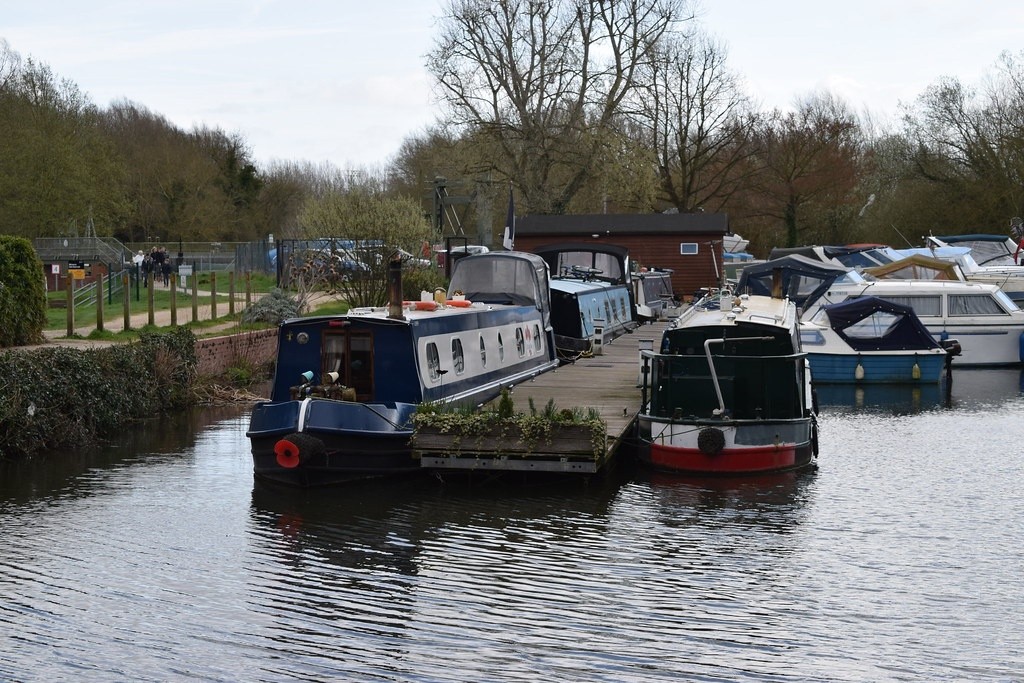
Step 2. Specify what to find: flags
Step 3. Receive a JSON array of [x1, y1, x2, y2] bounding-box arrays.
[[1013, 236, 1024, 264], [503, 186, 515, 251]]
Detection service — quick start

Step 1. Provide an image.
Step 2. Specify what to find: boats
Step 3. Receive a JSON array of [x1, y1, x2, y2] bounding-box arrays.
[[248, 252, 562, 481], [723, 234, 1024, 380], [631, 262, 679, 322], [532, 242, 635, 355], [639, 295, 819, 472]]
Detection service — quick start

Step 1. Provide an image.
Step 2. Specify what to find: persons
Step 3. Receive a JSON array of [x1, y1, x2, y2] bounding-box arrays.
[[133, 245, 171, 288]]
